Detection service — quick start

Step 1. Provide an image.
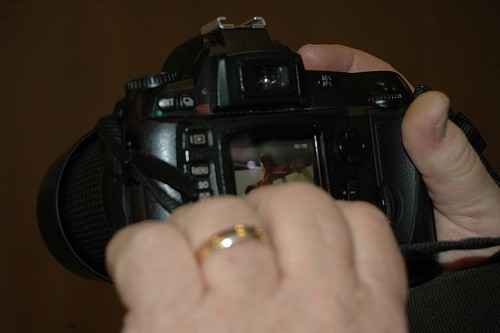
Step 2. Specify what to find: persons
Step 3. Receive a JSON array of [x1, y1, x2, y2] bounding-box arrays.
[[256, 152, 285, 187], [104, 42, 500, 333]]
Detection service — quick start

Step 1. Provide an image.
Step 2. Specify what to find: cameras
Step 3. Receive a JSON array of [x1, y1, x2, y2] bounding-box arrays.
[[36, 15, 454, 297]]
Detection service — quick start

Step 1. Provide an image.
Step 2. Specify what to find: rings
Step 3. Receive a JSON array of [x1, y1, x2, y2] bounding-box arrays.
[[194, 223, 274, 270]]
